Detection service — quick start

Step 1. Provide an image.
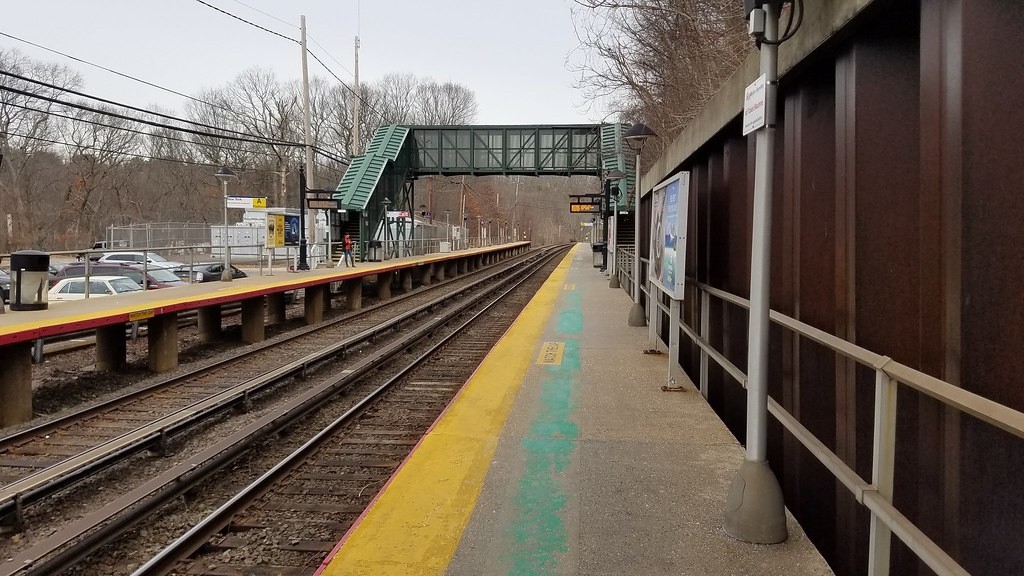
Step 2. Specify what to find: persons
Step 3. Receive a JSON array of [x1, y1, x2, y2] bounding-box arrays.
[[342, 232, 356, 267]]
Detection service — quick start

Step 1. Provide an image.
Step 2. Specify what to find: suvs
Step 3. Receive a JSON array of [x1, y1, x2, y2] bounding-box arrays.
[[76, 240, 129, 262], [49, 261, 190, 289]]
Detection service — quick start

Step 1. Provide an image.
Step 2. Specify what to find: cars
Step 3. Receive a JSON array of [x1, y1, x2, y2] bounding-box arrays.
[[173, 262, 246, 285], [96, 252, 169, 261], [48, 276, 144, 306], [0, 269, 11, 305]]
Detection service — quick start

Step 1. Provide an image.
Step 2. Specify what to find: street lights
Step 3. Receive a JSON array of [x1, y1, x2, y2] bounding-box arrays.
[[418, 204, 426, 254], [380, 196, 392, 260], [213, 165, 237, 283], [619, 122, 659, 326], [606, 169, 627, 288], [444, 209, 451, 241], [461, 212, 520, 250], [589, 214, 597, 248]]
